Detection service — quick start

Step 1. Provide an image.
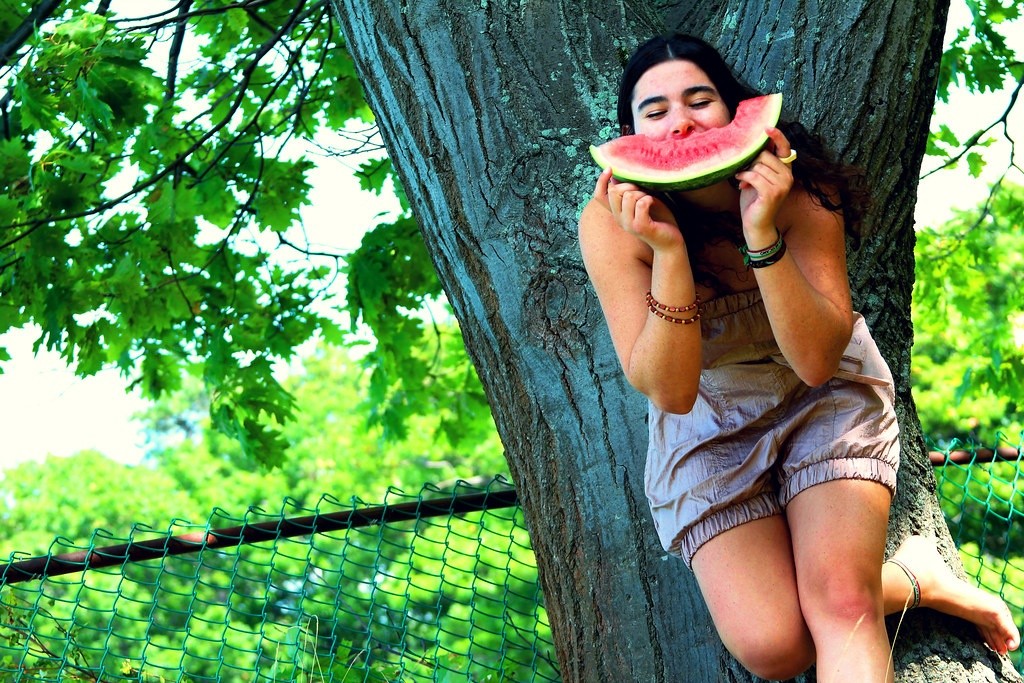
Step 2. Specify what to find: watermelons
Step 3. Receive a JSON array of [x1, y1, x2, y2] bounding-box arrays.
[[590, 92, 782, 192]]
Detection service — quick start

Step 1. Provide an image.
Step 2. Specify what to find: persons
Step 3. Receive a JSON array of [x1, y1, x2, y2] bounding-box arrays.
[[577, 32, 1021, 683]]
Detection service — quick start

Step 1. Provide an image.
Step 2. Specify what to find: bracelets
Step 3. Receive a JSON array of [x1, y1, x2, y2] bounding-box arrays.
[[647, 289, 701, 312], [645, 299, 703, 324], [738, 226, 787, 272]]
